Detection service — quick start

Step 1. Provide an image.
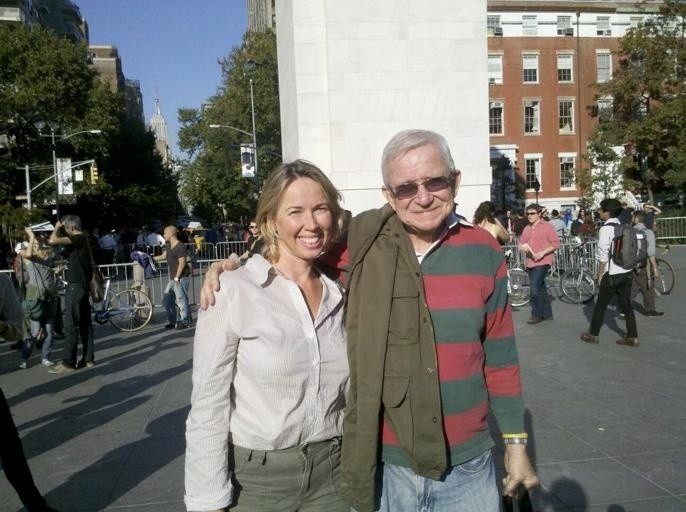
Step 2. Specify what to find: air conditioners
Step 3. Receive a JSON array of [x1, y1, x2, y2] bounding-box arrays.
[[563, 28, 573, 35], [493, 26, 503, 35], [490, 101, 503, 109], [525, 101, 533, 108], [604, 29, 611, 34]]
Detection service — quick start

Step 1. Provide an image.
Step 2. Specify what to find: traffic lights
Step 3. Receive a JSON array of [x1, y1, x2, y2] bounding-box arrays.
[[90, 166, 99, 185]]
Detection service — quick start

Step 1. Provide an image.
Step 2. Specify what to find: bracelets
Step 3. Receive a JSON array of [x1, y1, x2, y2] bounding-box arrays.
[[503, 438, 528, 445]]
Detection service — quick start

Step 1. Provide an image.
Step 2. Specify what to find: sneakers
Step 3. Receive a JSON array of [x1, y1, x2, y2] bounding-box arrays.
[[647, 311, 664, 317], [20, 358, 95, 373], [528, 315, 553, 324], [581, 332, 599, 344], [616, 337, 638, 346]]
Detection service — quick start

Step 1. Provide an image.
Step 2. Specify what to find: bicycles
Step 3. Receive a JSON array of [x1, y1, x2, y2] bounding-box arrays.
[[51, 268, 154, 334], [650, 253, 678, 295], [560, 240, 602, 306], [506, 244, 582, 308]]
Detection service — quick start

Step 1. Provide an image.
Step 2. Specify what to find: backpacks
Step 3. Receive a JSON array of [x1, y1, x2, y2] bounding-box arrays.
[[602, 222, 647, 270]]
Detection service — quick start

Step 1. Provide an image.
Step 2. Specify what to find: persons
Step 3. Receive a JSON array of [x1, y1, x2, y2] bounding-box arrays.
[[152, 225, 193, 330], [93, 222, 166, 278], [196, 132, 540, 512], [14, 215, 100, 374], [183, 161, 350, 511], [183, 220, 265, 268], [473, 201, 666, 348]]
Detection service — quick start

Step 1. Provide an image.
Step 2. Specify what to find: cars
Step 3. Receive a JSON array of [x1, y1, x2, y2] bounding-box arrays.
[[98, 214, 251, 262]]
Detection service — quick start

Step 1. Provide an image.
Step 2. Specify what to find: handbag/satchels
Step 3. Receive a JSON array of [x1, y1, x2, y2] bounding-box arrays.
[[90, 272, 103, 302], [22, 298, 43, 320]]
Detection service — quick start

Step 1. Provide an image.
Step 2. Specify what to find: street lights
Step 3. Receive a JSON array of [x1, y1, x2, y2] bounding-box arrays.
[[535, 177, 541, 205], [209, 122, 266, 223], [51, 124, 103, 183]]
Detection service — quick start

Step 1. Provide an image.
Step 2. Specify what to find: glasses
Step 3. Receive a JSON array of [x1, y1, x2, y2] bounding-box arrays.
[[526, 213, 535, 215], [388, 171, 449, 199]]
